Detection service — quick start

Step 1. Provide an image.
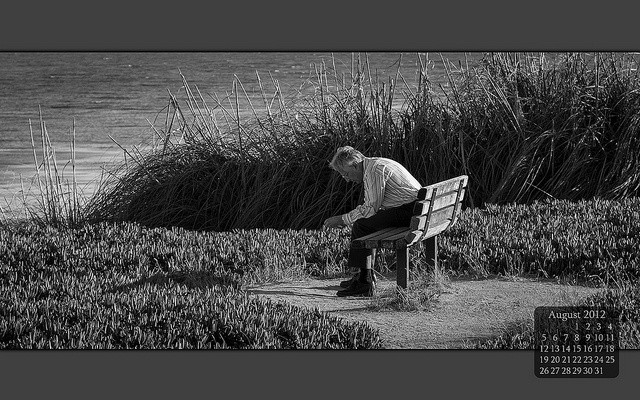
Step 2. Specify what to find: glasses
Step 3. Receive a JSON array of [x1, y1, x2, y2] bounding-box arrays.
[[342, 167, 352, 179]]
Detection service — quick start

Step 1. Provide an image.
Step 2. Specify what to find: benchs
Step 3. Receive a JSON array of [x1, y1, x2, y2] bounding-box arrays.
[[351, 175, 468, 305]]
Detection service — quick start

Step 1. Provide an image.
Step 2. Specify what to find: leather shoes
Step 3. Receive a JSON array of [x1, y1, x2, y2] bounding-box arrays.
[[336, 279, 373, 297], [340, 272, 377, 289]]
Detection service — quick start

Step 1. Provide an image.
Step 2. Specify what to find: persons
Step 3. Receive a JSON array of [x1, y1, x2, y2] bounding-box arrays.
[[322, 146, 421, 297]]
